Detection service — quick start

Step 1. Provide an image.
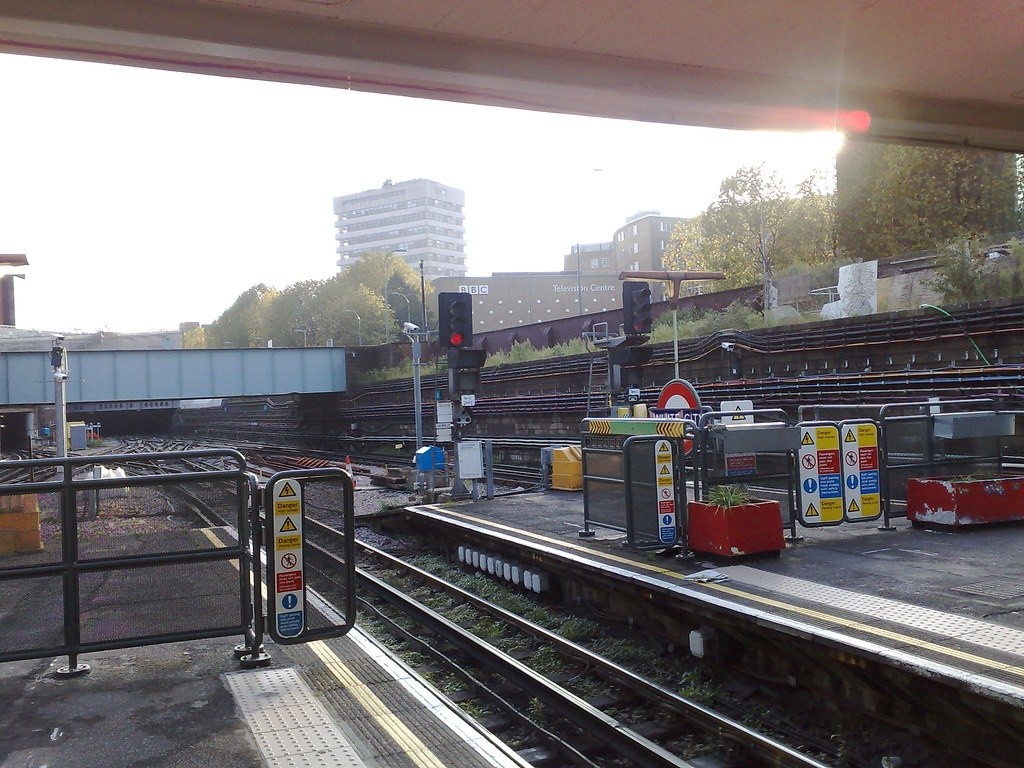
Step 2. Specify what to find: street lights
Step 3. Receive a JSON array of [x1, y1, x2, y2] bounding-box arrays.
[[295, 329, 307, 348], [344, 309, 362, 344], [390, 292, 410, 343], [385, 248, 408, 344]]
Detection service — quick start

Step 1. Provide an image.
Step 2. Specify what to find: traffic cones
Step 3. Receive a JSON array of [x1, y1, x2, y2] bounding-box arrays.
[[344, 454, 357, 488]]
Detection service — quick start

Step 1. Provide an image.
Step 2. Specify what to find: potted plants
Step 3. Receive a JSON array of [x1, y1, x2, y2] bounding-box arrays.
[[687, 483, 787, 557], [906, 474, 1024, 532]]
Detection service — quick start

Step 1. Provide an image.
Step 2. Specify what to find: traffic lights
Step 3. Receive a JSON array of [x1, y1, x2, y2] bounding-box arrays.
[[438, 292, 473, 348], [622, 281, 652, 334]]
